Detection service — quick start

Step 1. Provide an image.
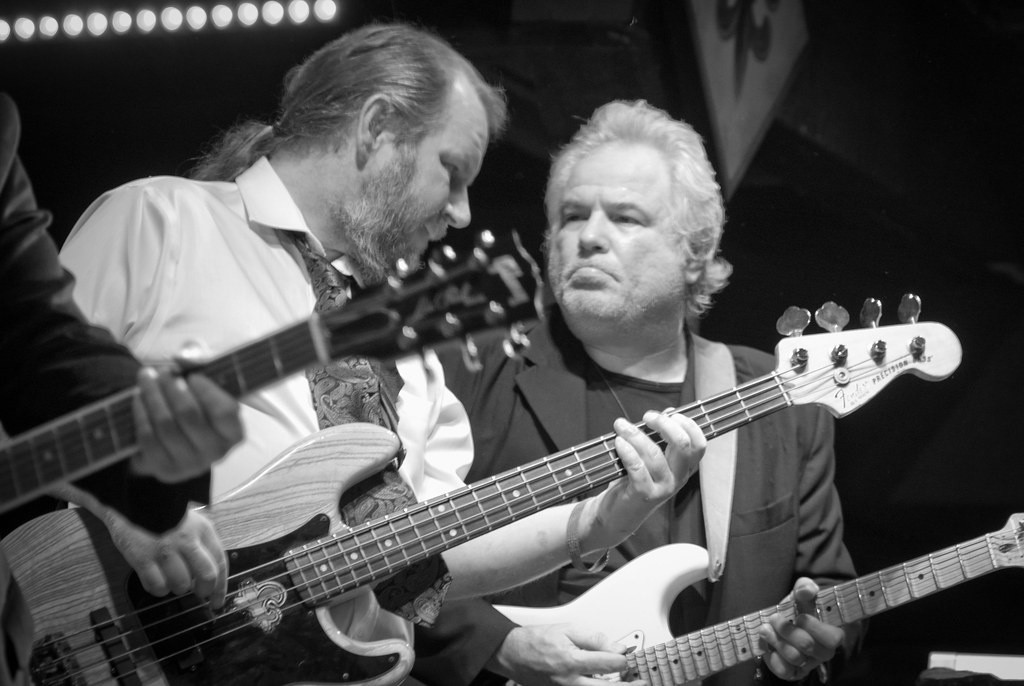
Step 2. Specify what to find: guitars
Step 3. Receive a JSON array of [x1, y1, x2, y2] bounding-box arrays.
[[491, 511, 1024, 686], [0, 294, 966, 686], [0, 227, 549, 515]]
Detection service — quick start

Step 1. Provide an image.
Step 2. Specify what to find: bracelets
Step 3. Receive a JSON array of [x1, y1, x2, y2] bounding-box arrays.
[[566, 496, 610, 576]]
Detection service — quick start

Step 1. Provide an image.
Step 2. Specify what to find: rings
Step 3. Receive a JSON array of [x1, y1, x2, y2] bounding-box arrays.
[[789, 667, 797, 680], [796, 657, 807, 667]]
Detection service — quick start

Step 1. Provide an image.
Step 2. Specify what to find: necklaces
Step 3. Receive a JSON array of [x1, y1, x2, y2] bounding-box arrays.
[[592, 362, 632, 423]]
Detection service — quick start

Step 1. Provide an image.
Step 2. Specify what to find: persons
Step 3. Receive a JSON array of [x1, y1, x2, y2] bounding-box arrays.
[[408, 99, 876, 686], [0, 96, 242, 686], [58, 23, 707, 686]]
